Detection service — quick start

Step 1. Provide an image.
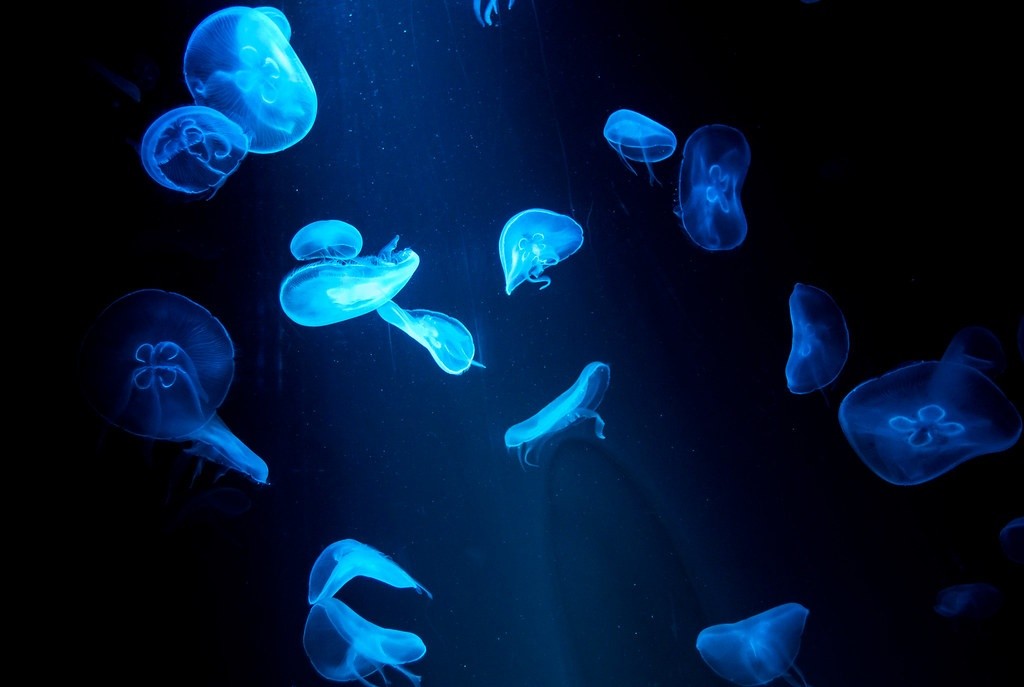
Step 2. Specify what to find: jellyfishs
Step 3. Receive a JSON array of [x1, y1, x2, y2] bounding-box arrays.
[[80, 0, 1023, 687]]
